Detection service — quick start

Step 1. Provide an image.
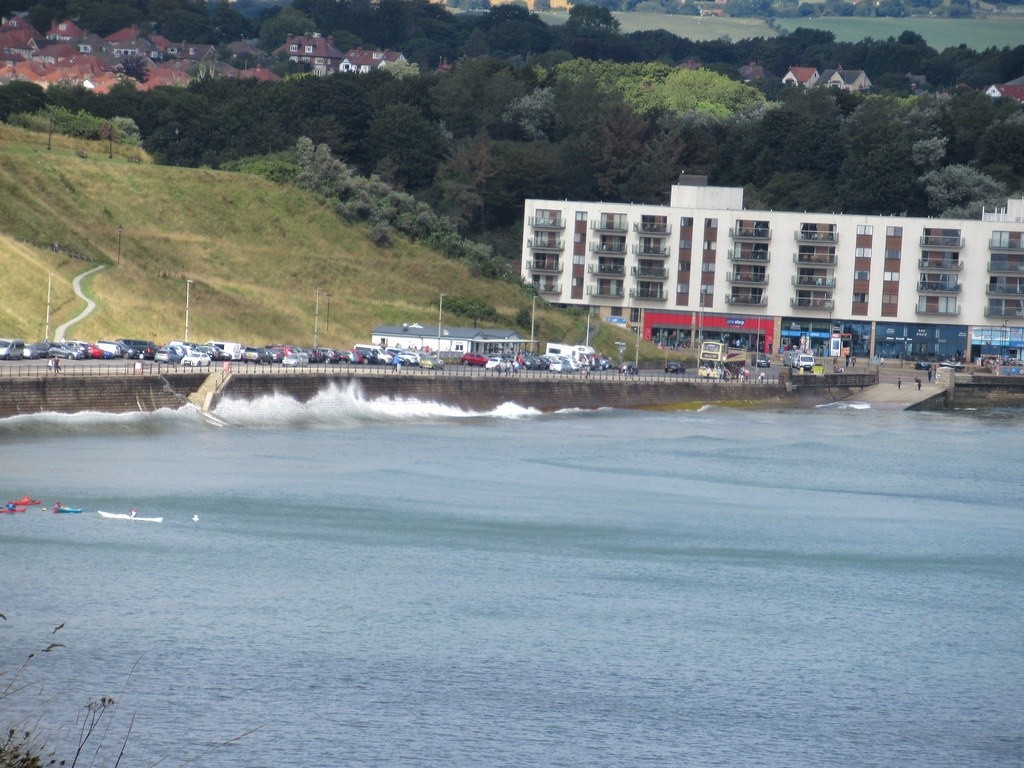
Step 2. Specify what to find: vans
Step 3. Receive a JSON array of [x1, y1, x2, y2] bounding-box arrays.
[[782, 351, 815, 370]]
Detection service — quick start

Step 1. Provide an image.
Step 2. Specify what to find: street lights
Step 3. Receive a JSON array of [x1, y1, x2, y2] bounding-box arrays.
[[313, 287, 322, 349], [1003, 317, 1009, 358], [45, 273, 54, 341], [437, 293, 447, 358], [696, 303, 704, 368], [586, 313, 594, 346], [530, 296, 538, 356], [184, 279, 194, 342]]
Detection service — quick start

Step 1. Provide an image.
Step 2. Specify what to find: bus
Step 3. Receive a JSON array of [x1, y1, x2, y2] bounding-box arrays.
[[695, 338, 748, 379]]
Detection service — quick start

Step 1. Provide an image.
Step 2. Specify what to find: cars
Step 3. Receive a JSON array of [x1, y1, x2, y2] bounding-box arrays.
[[483, 351, 615, 375], [0, 338, 446, 369], [915, 362, 931, 370], [750, 353, 771, 368], [618, 361, 634, 375], [664, 361, 686, 374], [460, 352, 490, 367]]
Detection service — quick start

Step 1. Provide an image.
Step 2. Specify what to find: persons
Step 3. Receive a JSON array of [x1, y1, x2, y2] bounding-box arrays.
[[0, 495, 137, 517], [585, 365, 591, 378], [392, 352, 399, 372], [496, 353, 523, 377], [914, 377, 921, 390], [845, 352, 884, 367], [759, 342, 798, 354], [897, 375, 902, 388], [928, 367, 932, 382], [48, 353, 61, 375], [621, 362, 765, 383], [985, 357, 1018, 376]]
